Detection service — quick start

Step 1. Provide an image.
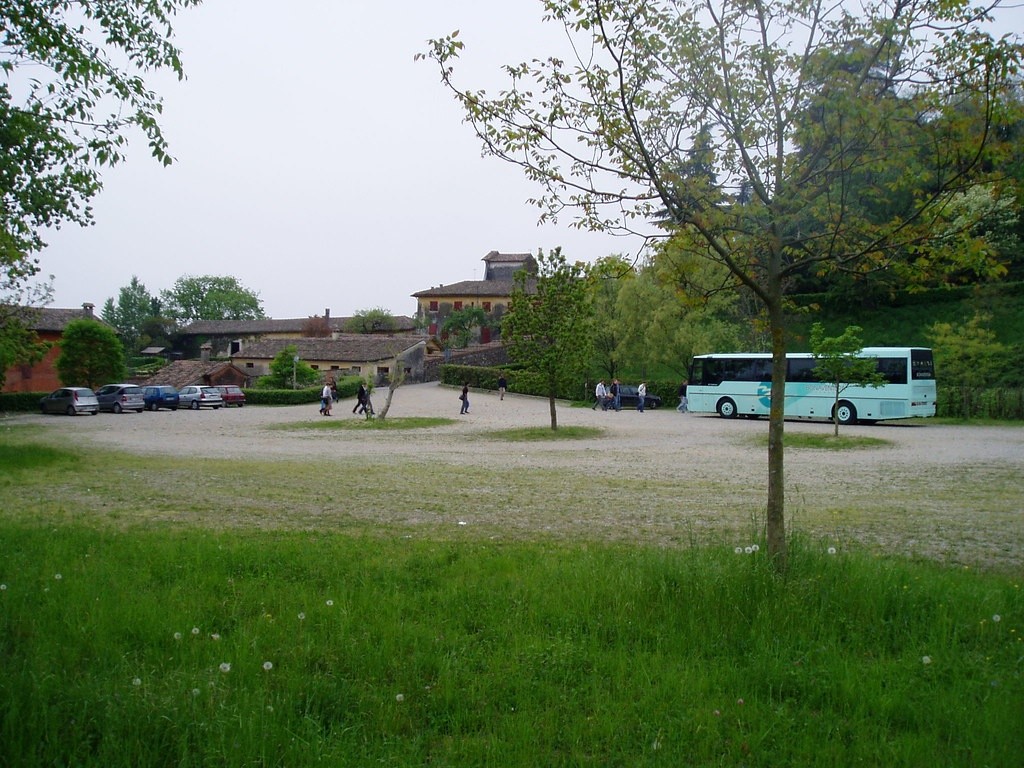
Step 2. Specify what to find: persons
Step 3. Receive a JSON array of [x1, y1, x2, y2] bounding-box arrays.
[[676, 379, 688, 413], [353, 383, 375, 415], [319, 377, 338, 416], [592, 379, 607, 411], [460, 382, 469, 414], [636, 382, 646, 413], [498, 373, 508, 400], [610, 378, 622, 412]]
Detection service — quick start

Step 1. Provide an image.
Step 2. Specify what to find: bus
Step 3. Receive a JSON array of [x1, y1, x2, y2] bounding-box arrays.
[[685, 346, 937, 426]]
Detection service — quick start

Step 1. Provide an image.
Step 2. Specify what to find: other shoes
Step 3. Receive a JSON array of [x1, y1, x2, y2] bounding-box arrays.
[[592, 407, 596, 411], [324, 413, 327, 415], [353, 411, 356, 414], [319, 410, 322, 415], [359, 412, 362, 415]]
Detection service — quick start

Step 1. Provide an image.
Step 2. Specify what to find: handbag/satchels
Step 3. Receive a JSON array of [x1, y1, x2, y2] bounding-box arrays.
[[608, 390, 613, 399], [459, 394, 463, 400]]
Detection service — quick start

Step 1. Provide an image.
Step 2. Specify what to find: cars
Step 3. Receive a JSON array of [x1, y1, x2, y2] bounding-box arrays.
[[39, 387, 100, 416], [619, 384, 662, 411], [93, 383, 145, 414], [212, 385, 247, 408], [176, 385, 223, 410], [140, 385, 180, 412]]
